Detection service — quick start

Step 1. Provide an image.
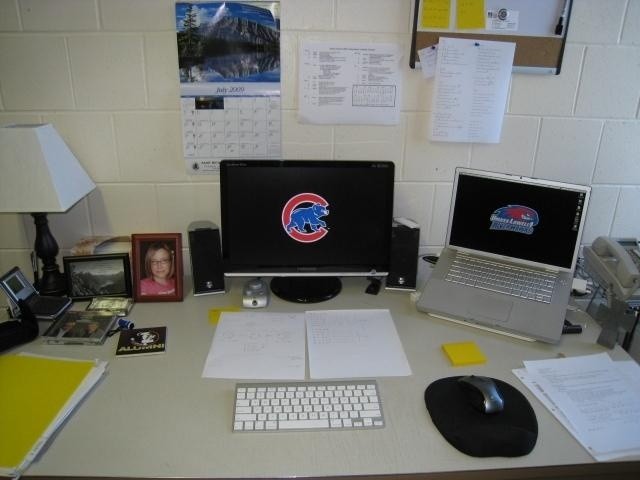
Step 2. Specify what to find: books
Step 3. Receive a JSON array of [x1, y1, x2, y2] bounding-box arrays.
[[115, 326, 166, 357], [86, 296, 133, 317], [40, 311, 118, 345]]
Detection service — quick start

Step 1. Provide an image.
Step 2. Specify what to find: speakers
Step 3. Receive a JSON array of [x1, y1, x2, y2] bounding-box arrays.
[[385, 217, 420, 292], [187, 220, 226, 297]]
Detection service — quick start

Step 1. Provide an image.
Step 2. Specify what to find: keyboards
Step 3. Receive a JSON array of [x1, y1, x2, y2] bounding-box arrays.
[[231, 380, 386, 434]]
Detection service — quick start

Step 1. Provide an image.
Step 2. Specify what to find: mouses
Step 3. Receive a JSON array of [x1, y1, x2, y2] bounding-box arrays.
[[457, 375, 505, 416]]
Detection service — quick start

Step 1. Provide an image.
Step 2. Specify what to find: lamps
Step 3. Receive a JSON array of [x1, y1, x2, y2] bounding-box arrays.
[[0, 123, 100, 298]]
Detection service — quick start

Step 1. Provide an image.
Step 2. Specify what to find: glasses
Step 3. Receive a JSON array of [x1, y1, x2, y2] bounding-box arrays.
[[151, 260, 168, 264]]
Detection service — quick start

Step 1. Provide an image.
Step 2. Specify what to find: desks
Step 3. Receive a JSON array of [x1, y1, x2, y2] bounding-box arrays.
[[2, 265, 638, 479]]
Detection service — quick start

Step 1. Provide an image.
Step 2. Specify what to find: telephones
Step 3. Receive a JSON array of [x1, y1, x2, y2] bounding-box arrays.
[[583, 236, 640, 300]]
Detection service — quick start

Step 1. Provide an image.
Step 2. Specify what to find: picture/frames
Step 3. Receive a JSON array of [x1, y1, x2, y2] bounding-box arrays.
[[61, 255, 134, 302], [130, 231, 183, 305]]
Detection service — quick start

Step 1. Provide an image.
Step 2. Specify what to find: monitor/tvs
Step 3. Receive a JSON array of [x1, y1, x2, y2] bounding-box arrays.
[[219, 159, 395, 304]]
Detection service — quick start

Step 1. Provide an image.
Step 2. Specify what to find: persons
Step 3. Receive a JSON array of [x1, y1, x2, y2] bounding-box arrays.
[[140, 242, 176, 296]]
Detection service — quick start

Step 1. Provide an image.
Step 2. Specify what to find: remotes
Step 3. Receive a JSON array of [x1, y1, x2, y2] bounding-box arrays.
[[394, 217, 420, 229]]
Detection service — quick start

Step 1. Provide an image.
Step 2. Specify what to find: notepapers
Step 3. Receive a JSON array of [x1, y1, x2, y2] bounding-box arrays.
[[442, 342, 487, 367]]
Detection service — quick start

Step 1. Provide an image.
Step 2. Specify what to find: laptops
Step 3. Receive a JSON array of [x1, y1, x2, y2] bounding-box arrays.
[[416, 167, 592, 346]]
